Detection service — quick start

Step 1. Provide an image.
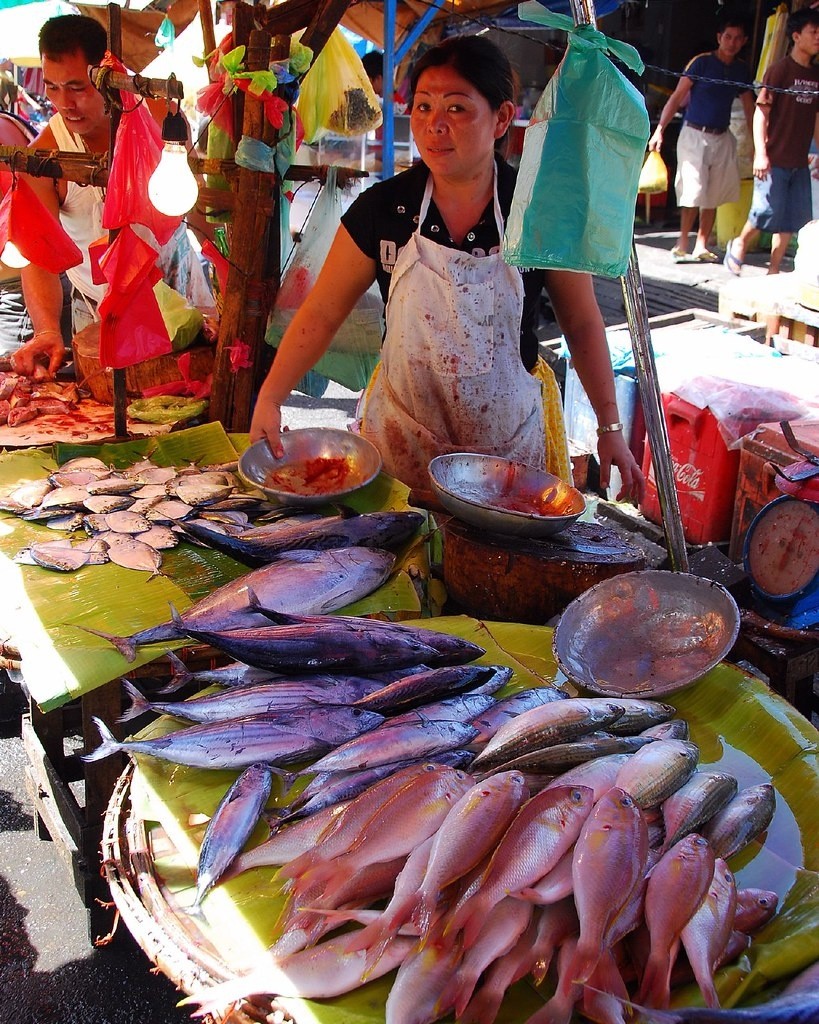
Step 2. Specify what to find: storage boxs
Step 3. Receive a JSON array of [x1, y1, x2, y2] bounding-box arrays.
[[562, 358, 647, 505], [728, 420, 819, 564], [637, 389, 803, 546]]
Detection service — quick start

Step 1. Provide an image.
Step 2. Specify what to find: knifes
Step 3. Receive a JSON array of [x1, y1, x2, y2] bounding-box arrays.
[[407, 487, 628, 556]]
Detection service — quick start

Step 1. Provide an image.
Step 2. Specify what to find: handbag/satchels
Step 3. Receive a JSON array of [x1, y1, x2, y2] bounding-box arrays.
[[638, 151, 668, 194], [264, 166, 386, 393], [297, 26, 383, 146]]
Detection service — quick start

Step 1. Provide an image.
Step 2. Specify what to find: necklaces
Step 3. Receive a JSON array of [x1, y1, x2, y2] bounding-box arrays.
[[81, 137, 91, 153]]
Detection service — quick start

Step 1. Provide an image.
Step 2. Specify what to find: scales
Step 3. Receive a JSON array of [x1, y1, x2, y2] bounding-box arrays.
[[743, 421, 819, 631]]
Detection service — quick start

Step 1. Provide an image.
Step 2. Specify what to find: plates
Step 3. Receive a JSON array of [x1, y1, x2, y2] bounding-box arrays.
[[551, 570, 740, 699], [426, 453, 588, 538], [237, 427, 382, 504], [742, 491, 819, 602]]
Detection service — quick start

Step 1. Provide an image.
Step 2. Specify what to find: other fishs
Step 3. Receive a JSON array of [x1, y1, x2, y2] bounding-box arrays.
[[0, 343, 93, 427], [61, 544, 398, 664], [0, 442, 247, 580], [147, 503, 426, 570], [78, 582, 819, 1024]]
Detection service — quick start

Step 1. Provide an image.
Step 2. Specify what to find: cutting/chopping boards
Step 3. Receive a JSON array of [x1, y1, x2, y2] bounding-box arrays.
[[435, 520, 651, 627], [71, 305, 220, 407]]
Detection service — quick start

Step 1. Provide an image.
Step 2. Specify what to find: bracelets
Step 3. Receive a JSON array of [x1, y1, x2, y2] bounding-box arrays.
[[597, 423, 623, 436], [35, 331, 60, 335]]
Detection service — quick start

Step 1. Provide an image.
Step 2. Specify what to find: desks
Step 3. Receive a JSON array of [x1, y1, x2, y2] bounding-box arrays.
[[717, 273, 819, 350]]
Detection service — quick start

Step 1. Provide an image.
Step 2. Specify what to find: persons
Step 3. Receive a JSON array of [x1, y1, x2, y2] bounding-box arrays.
[[647, 14, 757, 264], [723, 6, 819, 276], [360, 51, 410, 161], [246, 35, 648, 505], [0, 15, 225, 380]]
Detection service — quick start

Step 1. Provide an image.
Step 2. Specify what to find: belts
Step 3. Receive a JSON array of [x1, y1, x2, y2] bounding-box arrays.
[[687, 122, 726, 135]]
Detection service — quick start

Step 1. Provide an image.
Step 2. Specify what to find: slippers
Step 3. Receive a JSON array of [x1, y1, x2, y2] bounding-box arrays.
[[694, 253, 720, 263], [671, 248, 685, 258], [724, 240, 741, 277]]
